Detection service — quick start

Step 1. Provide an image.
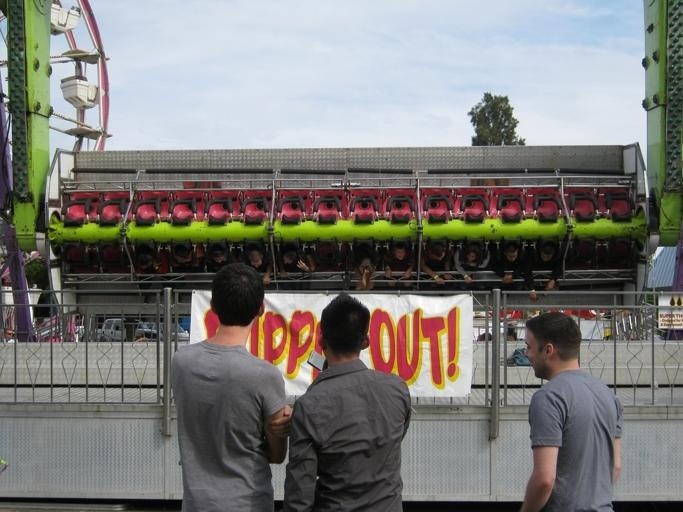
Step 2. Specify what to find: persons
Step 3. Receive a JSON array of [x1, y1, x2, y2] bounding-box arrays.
[[170, 262, 293, 512], [57, 242, 562, 301], [34, 286, 57, 326], [285, 295, 411, 510], [518, 312, 621, 511]]
